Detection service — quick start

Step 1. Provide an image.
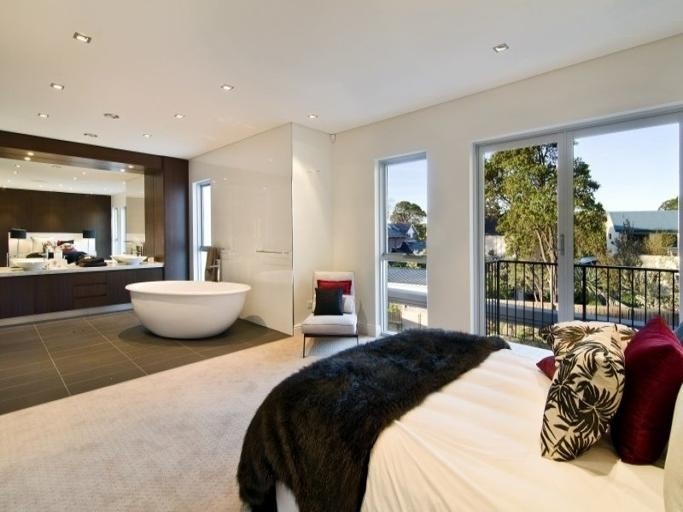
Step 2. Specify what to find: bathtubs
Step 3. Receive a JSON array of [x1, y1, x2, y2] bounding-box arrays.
[[124, 280, 253, 339]]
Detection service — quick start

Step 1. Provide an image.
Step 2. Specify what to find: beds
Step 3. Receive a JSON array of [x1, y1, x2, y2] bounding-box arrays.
[[6, 233, 98, 265], [237, 328, 682, 512]]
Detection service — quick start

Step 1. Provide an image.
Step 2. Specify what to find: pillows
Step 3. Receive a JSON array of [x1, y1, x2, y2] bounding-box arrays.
[[311, 280, 354, 316], [29, 236, 75, 254], [535, 313, 683, 464]]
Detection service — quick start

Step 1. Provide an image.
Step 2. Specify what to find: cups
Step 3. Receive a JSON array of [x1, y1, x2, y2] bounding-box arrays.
[[148, 256, 154, 264]]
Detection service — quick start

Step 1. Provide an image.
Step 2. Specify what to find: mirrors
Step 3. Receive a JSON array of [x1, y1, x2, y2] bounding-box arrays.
[[0, 148, 157, 267]]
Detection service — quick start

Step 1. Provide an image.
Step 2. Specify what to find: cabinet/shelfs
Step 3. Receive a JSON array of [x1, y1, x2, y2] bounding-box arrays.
[[0, 267, 165, 327]]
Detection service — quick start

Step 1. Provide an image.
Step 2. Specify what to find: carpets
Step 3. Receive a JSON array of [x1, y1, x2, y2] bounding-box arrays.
[[1, 335, 399, 512]]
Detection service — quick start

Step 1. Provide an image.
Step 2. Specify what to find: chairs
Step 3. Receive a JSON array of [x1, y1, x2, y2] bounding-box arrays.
[[301, 270, 360, 358]]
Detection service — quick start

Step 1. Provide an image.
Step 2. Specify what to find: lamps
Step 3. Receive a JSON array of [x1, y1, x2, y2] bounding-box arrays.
[[83, 228, 97, 256], [10, 227, 26, 258]]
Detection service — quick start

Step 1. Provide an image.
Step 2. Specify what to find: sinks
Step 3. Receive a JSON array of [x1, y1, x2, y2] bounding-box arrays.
[[109, 255, 147, 266], [9, 257, 50, 271]]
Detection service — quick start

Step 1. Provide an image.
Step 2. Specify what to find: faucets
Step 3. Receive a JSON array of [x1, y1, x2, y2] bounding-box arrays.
[[40, 247, 49, 259], [208, 259, 222, 282], [130, 248, 134, 254], [135, 246, 143, 256]]
